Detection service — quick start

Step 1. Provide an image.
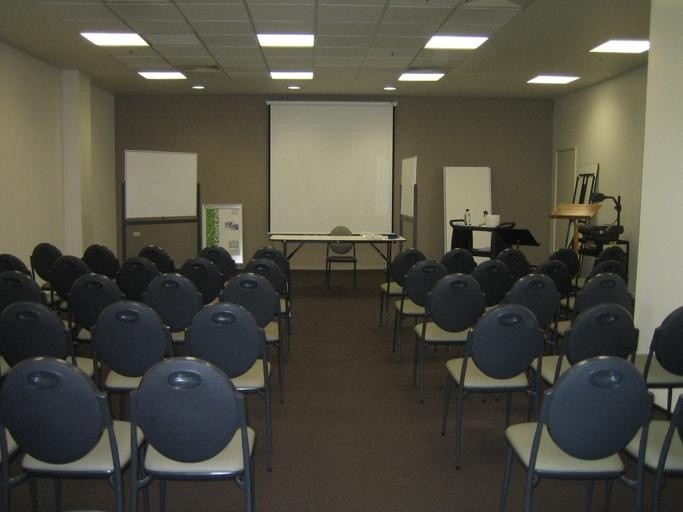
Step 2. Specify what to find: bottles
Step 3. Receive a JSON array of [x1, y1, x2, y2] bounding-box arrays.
[[463, 209, 470, 226]]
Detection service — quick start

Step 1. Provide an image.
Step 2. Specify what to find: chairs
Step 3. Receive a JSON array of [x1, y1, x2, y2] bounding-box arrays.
[[325, 226, 357, 291]]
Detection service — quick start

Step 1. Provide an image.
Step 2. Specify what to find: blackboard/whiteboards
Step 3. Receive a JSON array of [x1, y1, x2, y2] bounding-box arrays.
[[399, 155, 418, 218], [124, 150, 198, 222]]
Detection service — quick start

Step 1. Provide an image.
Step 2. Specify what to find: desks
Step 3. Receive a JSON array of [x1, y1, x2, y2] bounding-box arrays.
[[270, 233, 407, 293]]
